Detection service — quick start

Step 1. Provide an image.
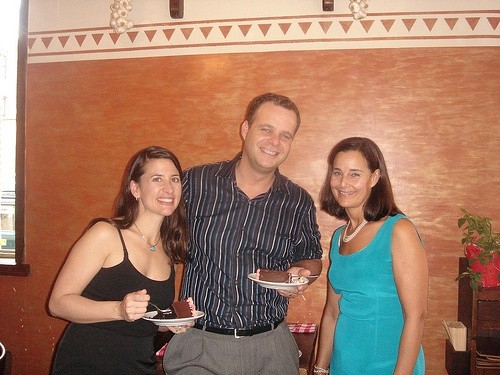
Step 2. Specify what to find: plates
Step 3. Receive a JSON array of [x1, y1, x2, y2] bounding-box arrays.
[[248, 273, 308, 291], [142, 310, 204, 326]]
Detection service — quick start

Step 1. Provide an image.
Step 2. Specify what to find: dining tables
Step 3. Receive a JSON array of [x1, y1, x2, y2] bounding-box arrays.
[[156, 322, 319, 375]]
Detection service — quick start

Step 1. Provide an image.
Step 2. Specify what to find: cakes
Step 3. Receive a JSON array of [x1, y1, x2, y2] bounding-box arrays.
[[151, 297, 196, 319], [255, 269, 292, 283]]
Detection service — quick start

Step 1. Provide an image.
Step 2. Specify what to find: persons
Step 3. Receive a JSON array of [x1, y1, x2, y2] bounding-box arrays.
[[313, 137, 429, 375], [49, 146, 195, 375], [163, 92, 323, 375]]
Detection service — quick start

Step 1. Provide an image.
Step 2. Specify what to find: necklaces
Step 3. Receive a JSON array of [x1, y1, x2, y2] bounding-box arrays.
[[343, 219, 368, 243], [133, 222, 162, 251]]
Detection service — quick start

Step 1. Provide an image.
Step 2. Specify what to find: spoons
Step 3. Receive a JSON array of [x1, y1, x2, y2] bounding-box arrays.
[[148, 302, 172, 314]]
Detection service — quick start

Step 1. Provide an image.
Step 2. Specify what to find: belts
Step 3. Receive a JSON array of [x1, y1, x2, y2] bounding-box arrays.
[[193, 317, 284, 337]]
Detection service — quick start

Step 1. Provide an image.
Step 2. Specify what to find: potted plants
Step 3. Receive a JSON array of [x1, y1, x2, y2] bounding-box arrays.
[[454, 206, 500, 292]]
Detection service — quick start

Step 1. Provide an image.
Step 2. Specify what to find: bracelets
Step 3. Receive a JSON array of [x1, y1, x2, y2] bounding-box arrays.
[[314, 365, 329, 375]]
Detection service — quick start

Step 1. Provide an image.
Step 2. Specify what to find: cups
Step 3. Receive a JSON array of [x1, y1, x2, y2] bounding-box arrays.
[[0, 342, 5, 375]]
